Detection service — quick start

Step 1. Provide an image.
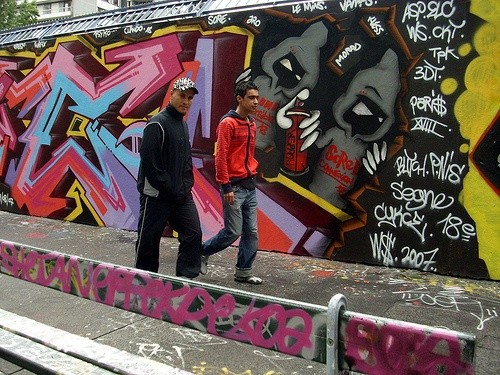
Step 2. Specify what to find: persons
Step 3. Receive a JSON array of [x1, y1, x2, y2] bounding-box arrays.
[[134, 78, 202, 281], [199, 81, 263, 284]]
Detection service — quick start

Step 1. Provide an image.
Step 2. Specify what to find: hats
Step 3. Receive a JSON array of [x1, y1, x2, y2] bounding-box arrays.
[[173, 78, 200, 94]]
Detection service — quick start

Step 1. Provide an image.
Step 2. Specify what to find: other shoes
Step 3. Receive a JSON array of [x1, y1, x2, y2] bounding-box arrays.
[[235, 272, 263, 283], [200, 250, 208, 274]]
